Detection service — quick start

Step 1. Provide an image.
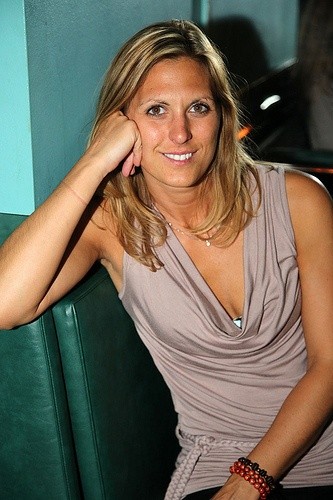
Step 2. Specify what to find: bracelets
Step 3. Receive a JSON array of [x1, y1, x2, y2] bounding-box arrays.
[[228, 457, 276, 500]]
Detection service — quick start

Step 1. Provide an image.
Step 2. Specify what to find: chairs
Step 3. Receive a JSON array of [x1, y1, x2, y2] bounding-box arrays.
[[0, 212, 79, 500], [53, 259, 181, 500]]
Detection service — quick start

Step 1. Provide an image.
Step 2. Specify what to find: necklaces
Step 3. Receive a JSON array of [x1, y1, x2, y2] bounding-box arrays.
[[167, 220, 218, 246]]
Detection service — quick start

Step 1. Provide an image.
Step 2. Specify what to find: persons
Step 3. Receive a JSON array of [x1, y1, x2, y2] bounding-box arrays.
[[0, 20, 333, 500]]
